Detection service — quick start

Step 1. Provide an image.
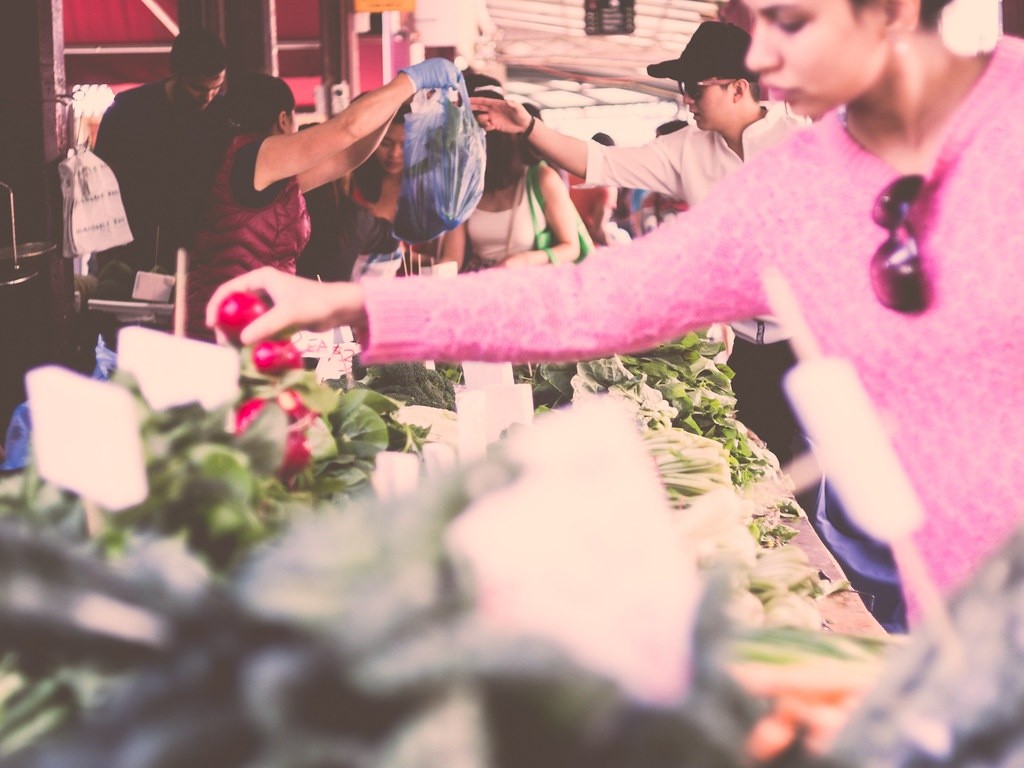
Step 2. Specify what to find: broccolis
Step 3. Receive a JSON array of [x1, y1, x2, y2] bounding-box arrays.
[[320, 362, 458, 414]]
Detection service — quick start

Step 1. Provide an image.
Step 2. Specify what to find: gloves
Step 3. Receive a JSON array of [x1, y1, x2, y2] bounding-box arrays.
[[400, 49, 463, 92]]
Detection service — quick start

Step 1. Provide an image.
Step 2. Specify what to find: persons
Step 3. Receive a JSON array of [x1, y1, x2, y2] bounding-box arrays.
[[643, 119, 698, 233], [208, 1, 1022, 631], [293, 122, 399, 283], [465, 21, 820, 468], [590, 131, 640, 238], [433, 71, 582, 271], [89, 23, 236, 300], [170, 52, 461, 347], [351, 99, 465, 278], [561, 168, 618, 247]]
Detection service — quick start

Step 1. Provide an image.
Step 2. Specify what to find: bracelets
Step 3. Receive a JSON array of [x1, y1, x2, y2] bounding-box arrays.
[[521, 115, 536, 136]]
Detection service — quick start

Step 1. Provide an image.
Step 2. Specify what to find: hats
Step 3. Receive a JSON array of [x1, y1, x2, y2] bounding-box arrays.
[[647, 22, 760, 88]]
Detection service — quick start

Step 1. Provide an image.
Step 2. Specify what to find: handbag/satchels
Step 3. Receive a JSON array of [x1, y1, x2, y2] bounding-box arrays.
[[515, 167, 592, 266], [391, 71, 486, 252]]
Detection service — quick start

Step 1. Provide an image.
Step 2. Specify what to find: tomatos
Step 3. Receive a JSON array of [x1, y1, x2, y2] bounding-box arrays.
[[234, 391, 322, 479], [250, 338, 303, 376], [215, 291, 269, 340]]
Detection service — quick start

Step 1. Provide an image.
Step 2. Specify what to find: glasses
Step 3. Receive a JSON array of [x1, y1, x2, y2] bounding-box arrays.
[[670, 75, 740, 103], [862, 168, 931, 319]]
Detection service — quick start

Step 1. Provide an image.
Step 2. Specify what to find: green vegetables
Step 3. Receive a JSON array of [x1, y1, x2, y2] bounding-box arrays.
[[512, 329, 808, 553]]
